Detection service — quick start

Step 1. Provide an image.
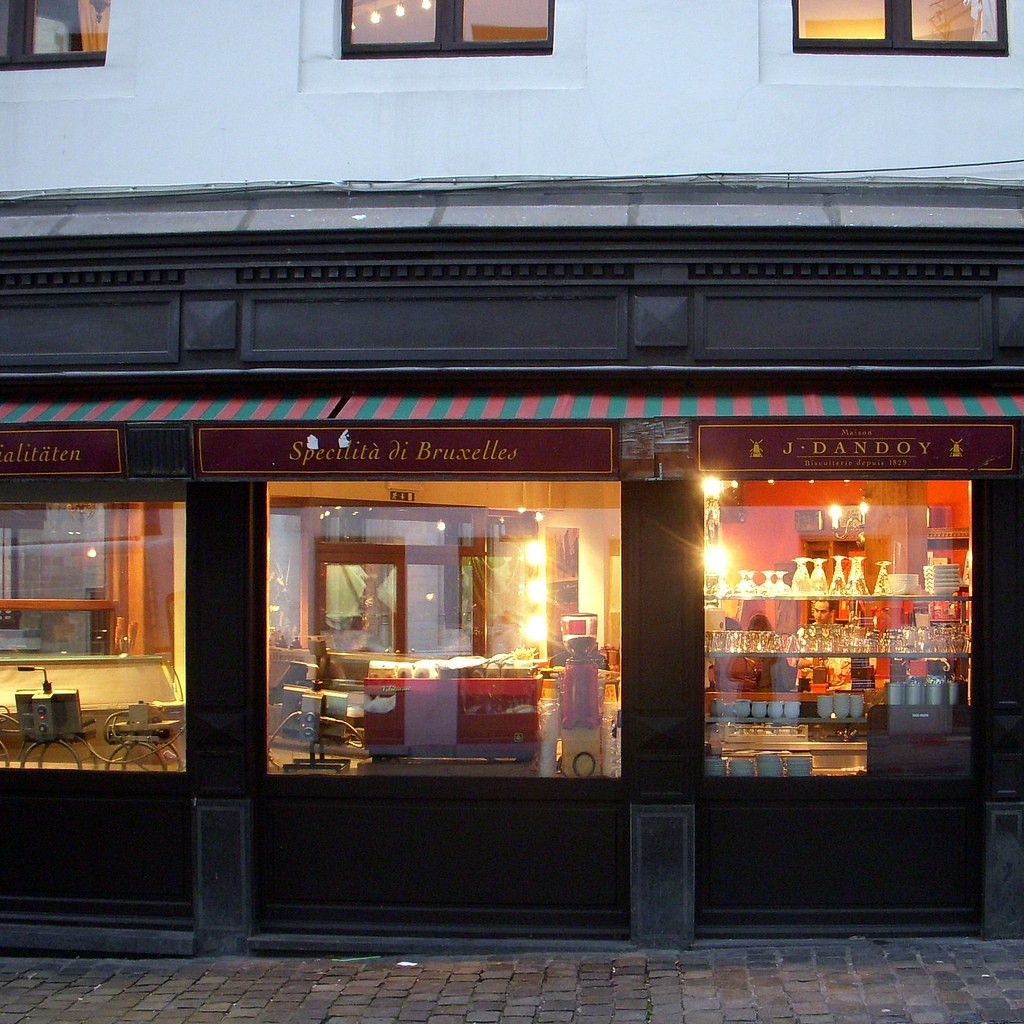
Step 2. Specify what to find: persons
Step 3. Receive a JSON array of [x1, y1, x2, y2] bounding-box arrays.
[[792, 599, 849, 718], [732, 614, 789, 692]]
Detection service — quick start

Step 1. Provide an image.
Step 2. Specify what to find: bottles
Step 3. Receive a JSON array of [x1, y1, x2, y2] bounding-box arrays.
[[710, 699, 723, 717], [115, 617, 126, 655]]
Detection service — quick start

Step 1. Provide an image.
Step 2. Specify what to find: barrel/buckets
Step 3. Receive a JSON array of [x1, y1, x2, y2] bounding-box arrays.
[[704, 758, 726, 777], [787, 758, 809, 776], [756, 756, 784, 777], [730, 759, 755, 777], [886, 682, 960, 706]]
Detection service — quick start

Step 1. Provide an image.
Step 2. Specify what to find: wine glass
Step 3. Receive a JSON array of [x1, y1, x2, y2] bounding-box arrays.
[[791, 557, 829, 595], [829, 556, 870, 595], [733, 571, 791, 596], [874, 561, 889, 595]]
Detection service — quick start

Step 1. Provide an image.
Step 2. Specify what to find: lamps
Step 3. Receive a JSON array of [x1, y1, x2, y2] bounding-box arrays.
[[828, 495, 869, 548], [351, 0, 432, 30], [90, 0, 111, 23]]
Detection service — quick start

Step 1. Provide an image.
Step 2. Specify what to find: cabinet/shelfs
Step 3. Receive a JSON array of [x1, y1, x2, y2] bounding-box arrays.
[[796, 533, 866, 685], [704, 592, 972, 724]]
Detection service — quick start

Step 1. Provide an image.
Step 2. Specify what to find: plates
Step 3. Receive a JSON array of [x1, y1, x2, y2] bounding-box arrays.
[[922, 564, 959, 595], [888, 574, 919, 594]]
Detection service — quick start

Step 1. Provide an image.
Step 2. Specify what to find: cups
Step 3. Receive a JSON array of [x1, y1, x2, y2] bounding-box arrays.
[[118, 639, 133, 658], [800, 624, 962, 653], [705, 630, 799, 653], [723, 699, 801, 719], [817, 694, 863, 719]]
